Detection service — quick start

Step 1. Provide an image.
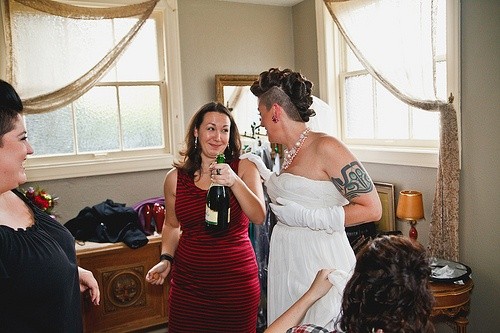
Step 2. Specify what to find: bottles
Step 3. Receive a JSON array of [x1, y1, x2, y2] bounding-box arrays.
[[205, 154, 230, 232]]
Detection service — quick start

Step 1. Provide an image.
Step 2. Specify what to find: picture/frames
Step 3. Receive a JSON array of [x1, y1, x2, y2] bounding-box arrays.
[[374, 182, 395, 231]]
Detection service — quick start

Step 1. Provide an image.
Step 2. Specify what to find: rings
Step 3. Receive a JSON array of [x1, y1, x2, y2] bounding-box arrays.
[[216, 169, 222, 175]]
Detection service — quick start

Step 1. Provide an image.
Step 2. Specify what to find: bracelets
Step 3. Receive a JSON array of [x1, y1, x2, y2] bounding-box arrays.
[[230, 177, 238, 188], [159, 254, 174, 265]]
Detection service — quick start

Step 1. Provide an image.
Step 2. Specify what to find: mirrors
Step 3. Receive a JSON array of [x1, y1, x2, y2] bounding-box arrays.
[[215, 75, 282, 176]]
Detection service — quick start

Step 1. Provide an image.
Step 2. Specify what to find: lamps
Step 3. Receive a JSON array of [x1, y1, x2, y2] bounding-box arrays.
[[395, 190, 424, 240]]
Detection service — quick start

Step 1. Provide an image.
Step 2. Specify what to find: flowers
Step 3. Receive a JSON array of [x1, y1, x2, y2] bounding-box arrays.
[[17, 182, 63, 220]]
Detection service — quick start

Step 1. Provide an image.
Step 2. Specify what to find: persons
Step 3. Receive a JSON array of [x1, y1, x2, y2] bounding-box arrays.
[[263, 236, 436, 333], [238, 66, 382, 327], [0, 78, 100, 333], [146, 101, 267, 333]]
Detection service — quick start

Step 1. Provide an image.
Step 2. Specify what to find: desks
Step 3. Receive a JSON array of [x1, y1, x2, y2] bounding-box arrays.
[[76, 232, 170, 333], [425, 257, 474, 333]]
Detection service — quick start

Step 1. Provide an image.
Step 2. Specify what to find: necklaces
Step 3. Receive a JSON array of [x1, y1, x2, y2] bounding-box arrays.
[[281, 128, 310, 169]]
